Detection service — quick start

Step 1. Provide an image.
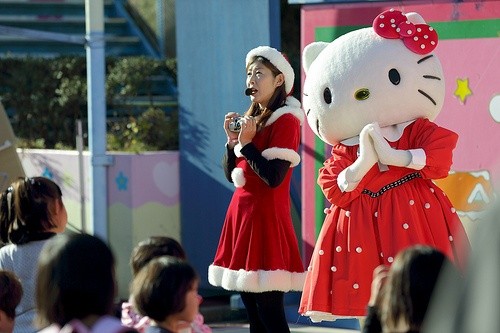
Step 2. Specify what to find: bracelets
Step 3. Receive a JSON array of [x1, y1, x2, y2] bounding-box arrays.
[[228, 138, 238, 143]]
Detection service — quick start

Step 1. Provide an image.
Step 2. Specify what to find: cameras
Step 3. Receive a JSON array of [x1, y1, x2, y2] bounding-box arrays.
[[228, 117, 246, 133]]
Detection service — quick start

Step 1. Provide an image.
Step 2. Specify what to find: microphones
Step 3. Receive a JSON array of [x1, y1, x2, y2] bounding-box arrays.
[[245, 88, 252, 96]]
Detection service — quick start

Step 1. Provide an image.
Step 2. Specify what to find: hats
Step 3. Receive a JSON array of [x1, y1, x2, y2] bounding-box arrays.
[[246, 46, 295, 96]]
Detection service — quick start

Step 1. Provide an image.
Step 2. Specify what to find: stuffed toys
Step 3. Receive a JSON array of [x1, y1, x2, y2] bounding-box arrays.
[[298, 9, 473, 333]]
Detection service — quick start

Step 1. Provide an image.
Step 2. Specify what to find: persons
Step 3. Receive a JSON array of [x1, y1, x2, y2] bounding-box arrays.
[[35, 232, 139, 333], [133, 255, 199, 333], [0, 177, 68, 333], [361, 244, 462, 333], [208, 45, 306, 333], [121, 236, 213, 333]]
